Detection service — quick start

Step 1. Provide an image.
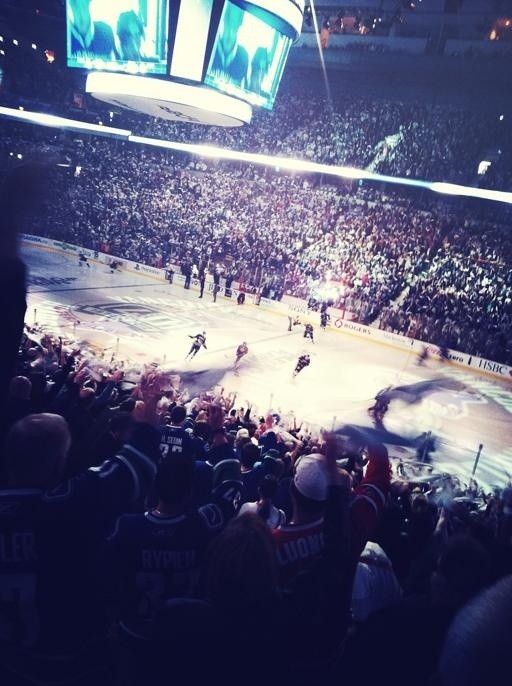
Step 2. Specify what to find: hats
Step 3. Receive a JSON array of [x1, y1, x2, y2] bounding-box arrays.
[[293, 453, 338, 508]]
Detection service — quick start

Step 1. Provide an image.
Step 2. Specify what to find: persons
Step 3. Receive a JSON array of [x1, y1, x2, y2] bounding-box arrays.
[[2, 88, 512, 685]]
